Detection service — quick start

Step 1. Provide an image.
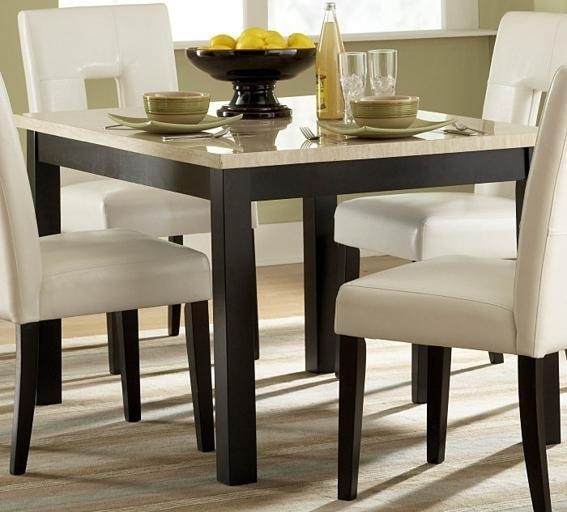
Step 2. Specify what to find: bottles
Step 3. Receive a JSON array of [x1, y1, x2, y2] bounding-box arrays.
[[315, 2, 348, 121]]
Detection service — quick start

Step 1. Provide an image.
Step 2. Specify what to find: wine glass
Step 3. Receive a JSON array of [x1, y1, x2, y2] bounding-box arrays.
[[185, 46, 316, 119]]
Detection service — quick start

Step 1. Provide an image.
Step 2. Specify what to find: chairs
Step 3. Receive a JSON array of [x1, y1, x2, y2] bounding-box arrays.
[[18, 3, 259, 376], [334, 67, 566, 511], [0, 73, 213, 476], [335, 11, 566, 410]]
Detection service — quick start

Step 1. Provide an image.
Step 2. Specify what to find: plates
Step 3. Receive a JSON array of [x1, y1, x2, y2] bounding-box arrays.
[[315, 118, 460, 140], [105, 112, 244, 135]]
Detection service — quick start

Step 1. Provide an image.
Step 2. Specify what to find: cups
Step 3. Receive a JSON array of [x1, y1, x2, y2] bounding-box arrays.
[[335, 48, 398, 123]]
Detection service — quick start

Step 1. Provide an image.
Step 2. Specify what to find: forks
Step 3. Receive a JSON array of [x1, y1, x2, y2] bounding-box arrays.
[[442, 120, 489, 137], [161, 124, 232, 140], [299, 126, 349, 144]]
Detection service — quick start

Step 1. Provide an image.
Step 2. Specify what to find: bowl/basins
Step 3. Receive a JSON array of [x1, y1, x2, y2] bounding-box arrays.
[[142, 91, 210, 124], [350, 95, 421, 128]]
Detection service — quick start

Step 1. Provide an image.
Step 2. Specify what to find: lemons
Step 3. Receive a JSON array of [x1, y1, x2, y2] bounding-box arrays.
[[197, 28, 316, 50]]
